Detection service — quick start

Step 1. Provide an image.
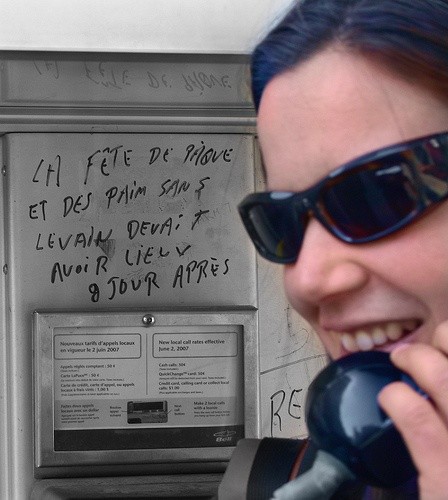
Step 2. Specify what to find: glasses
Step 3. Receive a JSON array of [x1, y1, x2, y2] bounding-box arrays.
[[237, 130, 448, 265]]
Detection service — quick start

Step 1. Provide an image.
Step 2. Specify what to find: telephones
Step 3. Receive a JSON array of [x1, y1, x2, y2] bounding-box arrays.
[[273, 351, 437, 500]]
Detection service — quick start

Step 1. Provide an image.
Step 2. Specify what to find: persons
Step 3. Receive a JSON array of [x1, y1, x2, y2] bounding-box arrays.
[[218, 0, 448, 500]]
